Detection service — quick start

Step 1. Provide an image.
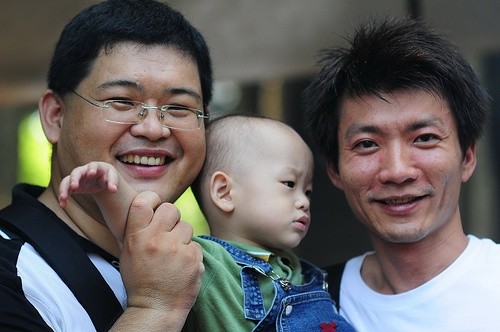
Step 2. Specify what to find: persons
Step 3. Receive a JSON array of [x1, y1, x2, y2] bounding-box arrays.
[[0, 0, 214, 332], [302, 13, 500, 332], [58, 113, 357, 332]]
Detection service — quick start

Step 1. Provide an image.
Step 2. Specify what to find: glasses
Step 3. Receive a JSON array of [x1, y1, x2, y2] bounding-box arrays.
[[69, 88, 211, 130]]
